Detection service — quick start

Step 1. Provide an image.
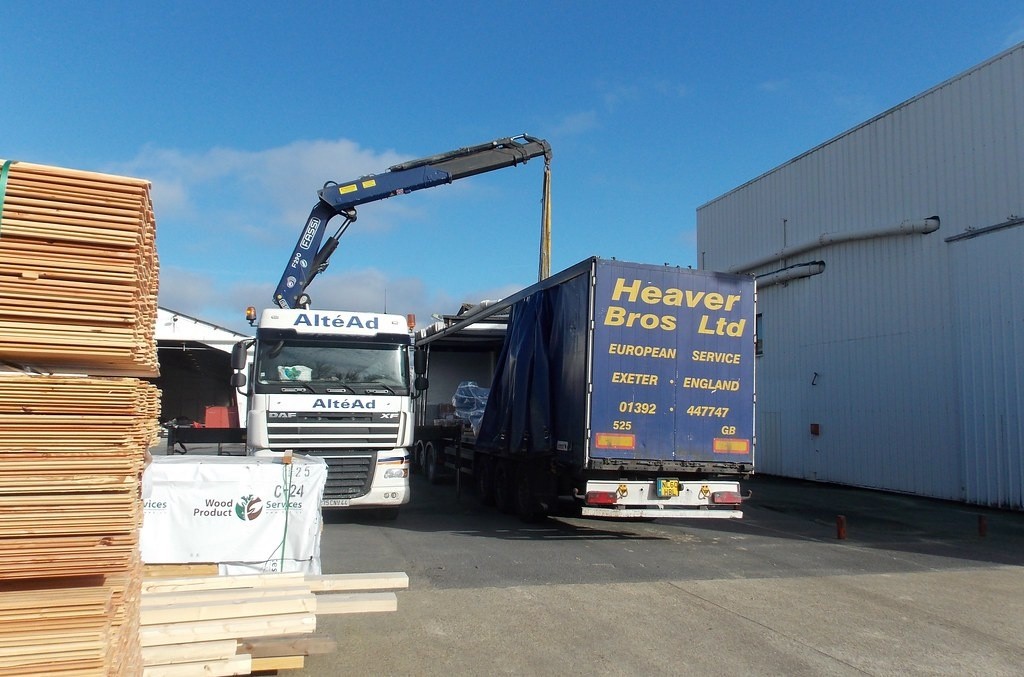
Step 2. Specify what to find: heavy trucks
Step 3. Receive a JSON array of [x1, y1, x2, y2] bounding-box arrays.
[[410, 256, 758, 521]]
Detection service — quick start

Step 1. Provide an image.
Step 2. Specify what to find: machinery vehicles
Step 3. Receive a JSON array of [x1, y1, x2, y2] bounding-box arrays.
[[166, 132, 552, 521]]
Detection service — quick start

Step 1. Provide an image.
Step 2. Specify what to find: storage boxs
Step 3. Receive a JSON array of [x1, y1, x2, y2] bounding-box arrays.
[[205, 406, 238, 428]]
